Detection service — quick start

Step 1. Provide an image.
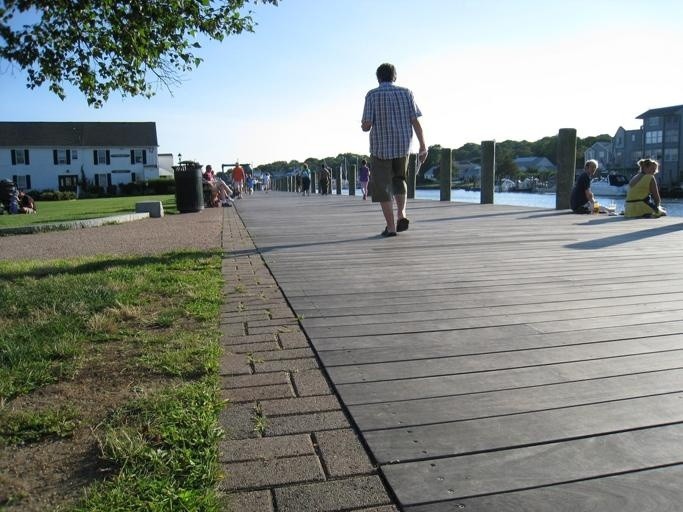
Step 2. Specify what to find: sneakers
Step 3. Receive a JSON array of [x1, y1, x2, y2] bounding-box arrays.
[[221, 192, 243, 207]]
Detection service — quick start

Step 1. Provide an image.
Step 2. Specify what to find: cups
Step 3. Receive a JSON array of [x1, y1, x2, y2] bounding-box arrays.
[[605, 196, 615, 216]]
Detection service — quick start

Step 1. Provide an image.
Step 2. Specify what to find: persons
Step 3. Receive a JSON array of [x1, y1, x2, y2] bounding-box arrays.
[[319, 163, 331, 196], [18, 192, 36, 214], [202, 161, 272, 208], [571, 159, 615, 214], [299, 163, 311, 197], [624, 157, 667, 218], [359, 159, 370, 200], [6, 190, 23, 214], [360, 62, 429, 237]]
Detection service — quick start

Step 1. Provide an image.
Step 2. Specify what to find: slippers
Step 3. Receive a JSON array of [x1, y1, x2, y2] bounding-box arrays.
[[382, 226, 396, 237], [396, 218, 409, 232]]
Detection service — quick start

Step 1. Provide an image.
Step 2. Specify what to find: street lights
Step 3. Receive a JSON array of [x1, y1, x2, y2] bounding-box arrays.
[[176, 152, 182, 166]]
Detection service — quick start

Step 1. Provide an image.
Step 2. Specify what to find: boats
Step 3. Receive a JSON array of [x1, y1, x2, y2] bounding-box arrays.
[[590, 173, 629, 196]]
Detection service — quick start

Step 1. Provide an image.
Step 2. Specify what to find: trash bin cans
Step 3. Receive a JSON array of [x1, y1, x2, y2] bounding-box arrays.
[[171, 161, 204, 214]]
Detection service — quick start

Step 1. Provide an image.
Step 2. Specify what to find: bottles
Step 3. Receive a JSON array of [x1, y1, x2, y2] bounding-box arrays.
[[592, 201, 599, 216]]
